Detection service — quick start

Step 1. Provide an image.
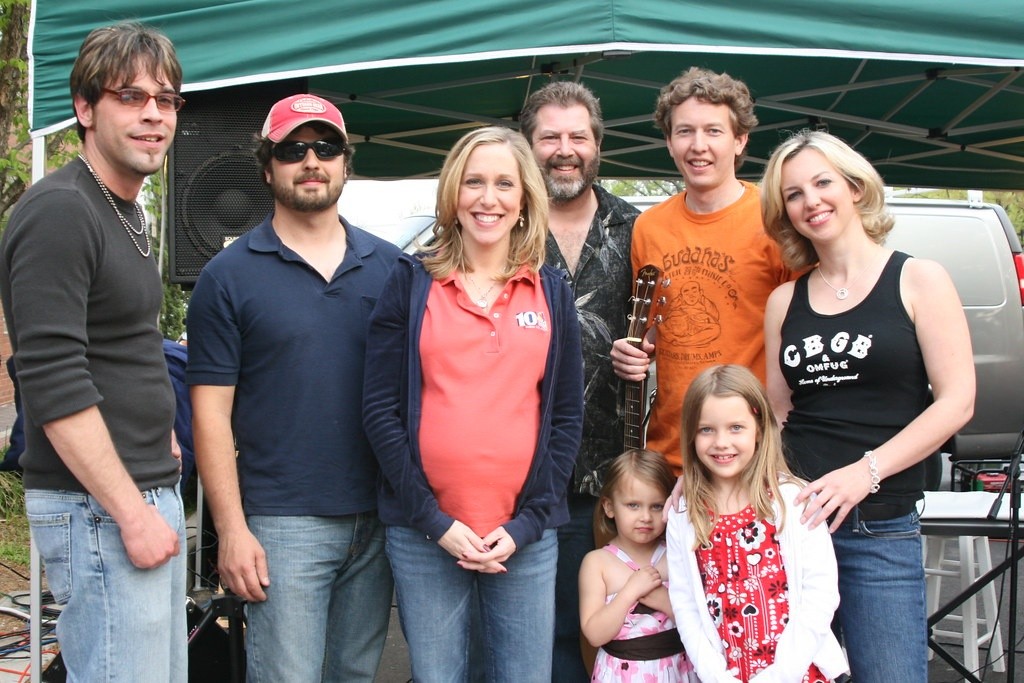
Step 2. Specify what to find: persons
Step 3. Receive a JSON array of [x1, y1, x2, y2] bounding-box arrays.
[[662, 131, 977, 683], [1, 24, 188, 682], [357, 127, 583, 683], [580, 447, 699, 683], [519, 80, 642, 683], [186, 95, 416, 682], [612, 63, 820, 488], [664, 362, 853, 683]]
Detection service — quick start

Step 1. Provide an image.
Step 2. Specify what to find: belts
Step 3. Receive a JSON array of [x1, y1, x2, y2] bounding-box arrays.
[[822, 500, 916, 523]]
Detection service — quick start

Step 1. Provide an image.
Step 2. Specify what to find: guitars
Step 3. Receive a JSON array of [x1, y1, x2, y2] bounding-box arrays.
[[577, 264, 672, 682]]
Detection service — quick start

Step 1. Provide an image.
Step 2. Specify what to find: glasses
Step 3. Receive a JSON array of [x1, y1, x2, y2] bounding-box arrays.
[[270, 138, 346, 162], [100, 87, 186, 112]]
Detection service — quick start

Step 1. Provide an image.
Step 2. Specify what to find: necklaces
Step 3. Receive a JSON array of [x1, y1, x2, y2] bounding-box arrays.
[[77, 152, 150, 257], [817, 243, 880, 300], [468, 265, 507, 308]]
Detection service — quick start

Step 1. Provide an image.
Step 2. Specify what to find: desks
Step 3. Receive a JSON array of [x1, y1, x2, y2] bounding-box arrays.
[[920, 517, 1024, 683]]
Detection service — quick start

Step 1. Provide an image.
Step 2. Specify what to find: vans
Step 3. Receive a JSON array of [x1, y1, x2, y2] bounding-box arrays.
[[378, 193, 1023, 470]]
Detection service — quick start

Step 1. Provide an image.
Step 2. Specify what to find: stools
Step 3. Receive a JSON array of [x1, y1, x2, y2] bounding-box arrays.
[[923, 535, 1006, 683]]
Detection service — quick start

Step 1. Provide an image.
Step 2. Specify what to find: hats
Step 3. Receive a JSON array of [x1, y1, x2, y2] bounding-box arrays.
[[261, 94, 348, 145]]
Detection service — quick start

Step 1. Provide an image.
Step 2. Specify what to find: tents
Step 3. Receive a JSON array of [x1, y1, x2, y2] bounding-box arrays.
[[30, 0, 1023, 683]]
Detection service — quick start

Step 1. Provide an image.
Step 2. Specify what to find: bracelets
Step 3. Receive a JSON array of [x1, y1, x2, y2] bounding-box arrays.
[[862, 450, 880, 493]]
